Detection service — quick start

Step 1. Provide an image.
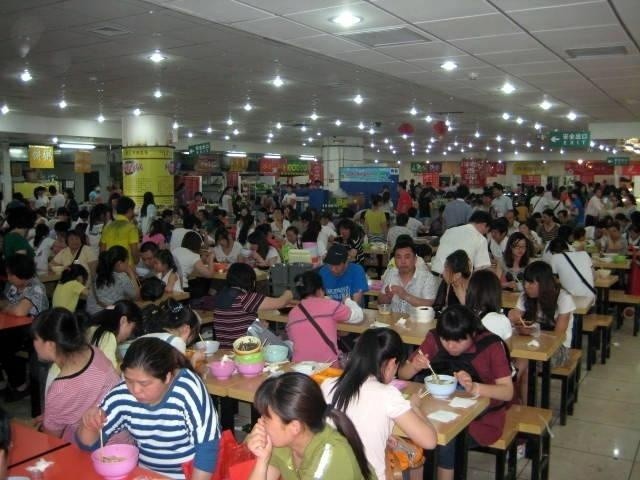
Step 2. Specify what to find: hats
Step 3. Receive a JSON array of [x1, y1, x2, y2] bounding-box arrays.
[[323, 244, 349, 265]]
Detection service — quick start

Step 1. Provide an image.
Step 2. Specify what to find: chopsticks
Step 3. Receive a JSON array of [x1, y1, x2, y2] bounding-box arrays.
[[99, 410, 103, 460], [198, 334, 205, 343], [418, 351, 437, 379], [518, 316, 527, 327], [422, 390, 432, 399]]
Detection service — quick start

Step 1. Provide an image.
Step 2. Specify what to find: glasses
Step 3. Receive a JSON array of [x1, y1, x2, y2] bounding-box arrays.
[[515, 246, 527, 249]]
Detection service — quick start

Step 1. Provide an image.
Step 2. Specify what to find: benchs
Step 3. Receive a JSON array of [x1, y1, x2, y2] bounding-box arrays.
[[518, 402, 554, 478], [601, 316, 615, 362], [583, 320, 600, 370], [531, 351, 582, 426], [486, 428, 513, 478], [607, 295, 640, 339]]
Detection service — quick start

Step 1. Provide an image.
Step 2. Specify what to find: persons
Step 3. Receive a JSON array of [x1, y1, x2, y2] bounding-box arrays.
[[386, 233, 429, 274], [48, 186, 65, 210], [527, 217, 541, 235], [242, 231, 283, 271], [504, 210, 520, 237], [51, 263, 89, 312], [328, 220, 366, 265], [465, 269, 514, 342], [352, 208, 368, 223], [631, 211, 640, 225], [107, 192, 123, 221], [557, 211, 576, 228], [419, 187, 433, 218], [382, 192, 394, 217], [177, 206, 190, 216], [600, 214, 613, 226], [404, 207, 428, 238], [33, 185, 49, 207], [284, 270, 353, 369], [430, 209, 492, 292], [37, 205, 89, 229], [394, 181, 413, 213], [136, 297, 208, 371], [1, 199, 37, 257], [139, 191, 159, 234], [169, 215, 208, 253], [72, 335, 221, 480], [84, 244, 139, 315], [490, 184, 514, 218], [158, 209, 176, 243], [584, 214, 597, 240], [152, 249, 185, 293], [544, 225, 577, 257], [63, 189, 79, 212], [320, 327, 438, 480], [130, 241, 159, 274], [301, 231, 328, 260], [49, 230, 97, 270], [615, 213, 631, 233], [283, 226, 303, 251], [141, 218, 167, 250], [379, 186, 390, 195], [246, 371, 377, 480], [50, 222, 71, 258], [175, 182, 189, 207], [0, 409, 30, 480], [315, 243, 370, 311], [43, 299, 142, 401], [98, 195, 141, 271], [505, 260, 577, 462], [364, 192, 388, 280], [377, 239, 439, 310], [547, 237, 598, 305], [188, 191, 204, 220], [415, 182, 423, 188], [431, 205, 445, 234], [519, 222, 540, 258], [486, 217, 511, 243], [211, 262, 294, 350], [573, 227, 587, 252], [12, 191, 35, 209], [386, 212, 418, 265], [601, 222, 629, 257], [31, 310, 137, 447], [417, 244, 433, 263], [28, 223, 53, 276], [441, 183, 474, 228], [626, 224, 640, 262], [89, 185, 102, 203], [539, 208, 561, 241], [399, 305, 516, 480], [407, 180, 415, 193], [198, 179, 339, 235], [529, 178, 640, 215], [431, 249, 475, 318], [171, 231, 214, 293], [211, 227, 243, 266], [84, 203, 109, 247], [489, 219, 511, 257], [501, 230, 531, 291], [0, 253, 52, 406]]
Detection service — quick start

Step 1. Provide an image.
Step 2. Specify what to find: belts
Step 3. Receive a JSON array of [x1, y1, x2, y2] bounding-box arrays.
[[431, 271, 443, 278]]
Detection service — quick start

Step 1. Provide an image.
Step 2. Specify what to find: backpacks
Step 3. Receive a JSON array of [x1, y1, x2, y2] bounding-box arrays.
[[430, 329, 511, 423]]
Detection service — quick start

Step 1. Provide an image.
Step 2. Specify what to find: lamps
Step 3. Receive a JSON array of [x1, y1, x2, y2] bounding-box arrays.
[[59, 142, 96, 150], [224, 153, 248, 158], [263, 154, 281, 160], [300, 156, 318, 162]]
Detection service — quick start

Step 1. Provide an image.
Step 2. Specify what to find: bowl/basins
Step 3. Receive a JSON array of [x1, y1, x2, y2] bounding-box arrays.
[[197, 341, 219, 356], [91, 444, 139, 480], [378, 303, 392, 315], [424, 373, 457, 399], [136, 268, 148, 276], [303, 242, 318, 263], [612, 256, 625, 263], [235, 352, 265, 376], [233, 336, 261, 354], [264, 345, 288, 363], [208, 362, 235, 380], [276, 306, 293, 314], [515, 321, 539, 335], [241, 249, 255, 257], [598, 268, 611, 279]]
